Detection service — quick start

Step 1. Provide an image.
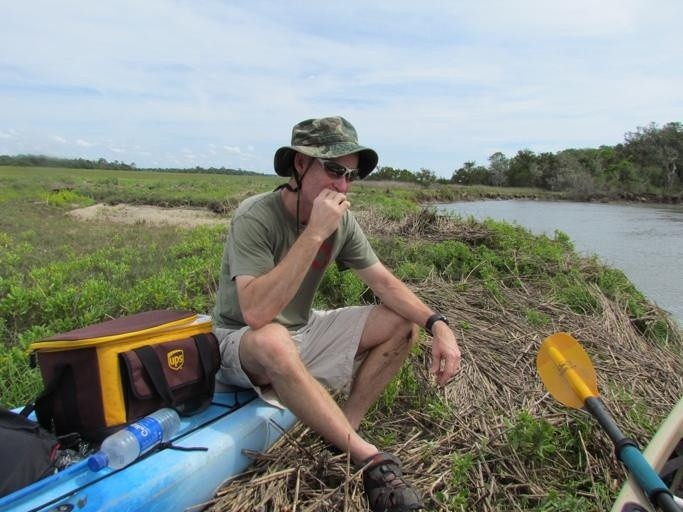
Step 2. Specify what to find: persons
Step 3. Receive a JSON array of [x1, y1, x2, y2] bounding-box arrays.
[[205, 115, 462, 511]]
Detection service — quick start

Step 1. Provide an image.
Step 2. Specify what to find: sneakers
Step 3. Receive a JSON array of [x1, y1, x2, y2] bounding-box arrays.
[[350, 453, 425, 512]]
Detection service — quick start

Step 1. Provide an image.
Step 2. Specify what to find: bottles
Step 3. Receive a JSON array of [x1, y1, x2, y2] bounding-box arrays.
[[87, 407, 181, 473]]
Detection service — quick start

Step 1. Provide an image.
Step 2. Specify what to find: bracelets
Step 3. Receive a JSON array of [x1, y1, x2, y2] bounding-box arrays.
[[425, 311, 449, 338]]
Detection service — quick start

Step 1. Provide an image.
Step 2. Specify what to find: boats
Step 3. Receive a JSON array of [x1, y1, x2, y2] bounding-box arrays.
[[1, 382, 297, 512]]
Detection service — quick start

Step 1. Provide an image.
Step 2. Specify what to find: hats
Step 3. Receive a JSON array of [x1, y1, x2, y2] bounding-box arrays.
[[275, 117, 378, 179]]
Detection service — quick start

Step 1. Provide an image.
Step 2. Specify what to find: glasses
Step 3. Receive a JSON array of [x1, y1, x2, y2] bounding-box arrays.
[[317, 158, 359, 181]]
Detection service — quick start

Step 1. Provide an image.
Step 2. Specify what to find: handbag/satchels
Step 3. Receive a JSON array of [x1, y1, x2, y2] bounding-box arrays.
[[30, 310, 222, 454]]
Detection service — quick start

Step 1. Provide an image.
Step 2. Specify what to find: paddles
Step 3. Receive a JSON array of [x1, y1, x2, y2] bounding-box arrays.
[[537, 333, 683, 511]]
